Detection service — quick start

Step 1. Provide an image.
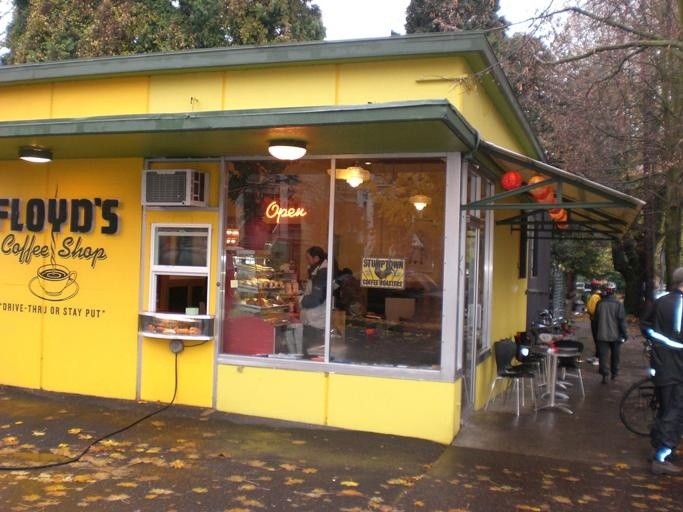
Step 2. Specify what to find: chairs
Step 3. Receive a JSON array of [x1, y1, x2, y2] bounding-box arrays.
[[484, 333, 587, 418]]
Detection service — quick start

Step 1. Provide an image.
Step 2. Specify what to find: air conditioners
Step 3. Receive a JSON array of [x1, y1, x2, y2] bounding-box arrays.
[[140, 168, 208, 208]]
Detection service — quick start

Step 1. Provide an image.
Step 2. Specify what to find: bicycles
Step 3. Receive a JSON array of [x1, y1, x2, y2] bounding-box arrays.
[[618, 336, 660, 437]]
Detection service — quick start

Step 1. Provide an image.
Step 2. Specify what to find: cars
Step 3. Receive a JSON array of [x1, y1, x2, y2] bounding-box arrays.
[[335, 269, 442, 324], [575, 282, 584, 290]]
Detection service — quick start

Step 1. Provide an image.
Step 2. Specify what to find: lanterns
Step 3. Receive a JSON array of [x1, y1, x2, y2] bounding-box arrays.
[[501, 168, 523, 189], [528, 175, 568, 229]]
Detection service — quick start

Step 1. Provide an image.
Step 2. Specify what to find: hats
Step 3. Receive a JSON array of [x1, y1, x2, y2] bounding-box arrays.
[[606, 282, 616, 291]]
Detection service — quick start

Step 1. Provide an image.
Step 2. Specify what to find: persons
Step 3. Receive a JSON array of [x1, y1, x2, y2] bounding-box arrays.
[[567, 276, 630, 384], [638, 266, 683, 475], [286, 247, 370, 363]]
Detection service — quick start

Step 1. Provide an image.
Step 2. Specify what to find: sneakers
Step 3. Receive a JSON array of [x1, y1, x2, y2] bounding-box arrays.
[[603, 372, 617, 383], [587, 356, 598, 367], [649, 451, 683, 475]]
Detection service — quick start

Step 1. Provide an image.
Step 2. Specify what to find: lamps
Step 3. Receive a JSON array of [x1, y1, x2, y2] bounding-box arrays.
[[345, 166, 373, 191], [408, 193, 432, 213], [270, 140, 307, 164]]
